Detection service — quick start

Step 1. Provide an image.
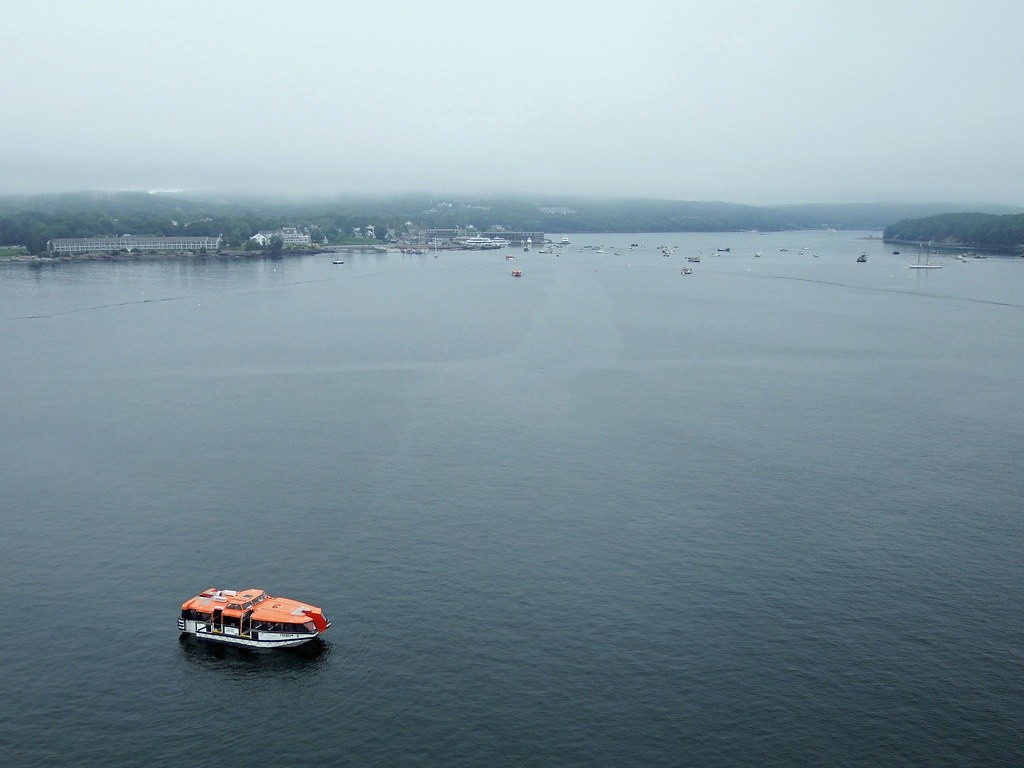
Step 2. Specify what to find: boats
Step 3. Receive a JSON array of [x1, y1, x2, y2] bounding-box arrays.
[[780, 246, 819, 258], [512, 269, 522, 276], [523, 236, 569, 254], [657, 244, 678, 259], [576, 242, 641, 255], [958, 250, 985, 264], [460, 236, 509, 249], [178, 588, 331, 650], [333, 258, 344, 264], [718, 247, 730, 251], [857, 252, 869, 262], [756, 249, 762, 257], [688, 254, 701, 262], [681, 265, 693, 274]]
